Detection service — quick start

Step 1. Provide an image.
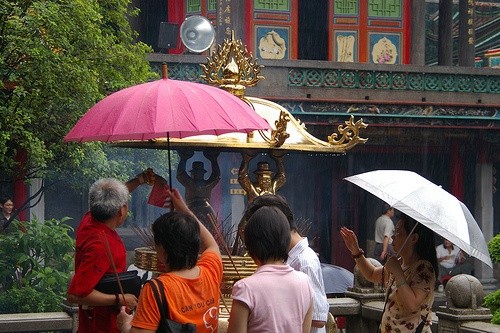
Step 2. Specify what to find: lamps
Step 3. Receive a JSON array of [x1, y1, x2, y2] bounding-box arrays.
[[180, 15, 215, 53]]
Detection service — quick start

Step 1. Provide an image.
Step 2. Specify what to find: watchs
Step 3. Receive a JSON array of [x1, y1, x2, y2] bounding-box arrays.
[[352, 249, 365, 261]]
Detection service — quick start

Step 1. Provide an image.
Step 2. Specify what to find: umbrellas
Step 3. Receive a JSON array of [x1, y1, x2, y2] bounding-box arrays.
[[321, 262, 355, 294], [344, 168, 494, 270], [63, 62, 273, 210]]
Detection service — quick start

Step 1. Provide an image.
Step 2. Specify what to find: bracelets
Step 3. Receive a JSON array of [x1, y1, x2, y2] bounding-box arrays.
[[115, 293, 121, 305]]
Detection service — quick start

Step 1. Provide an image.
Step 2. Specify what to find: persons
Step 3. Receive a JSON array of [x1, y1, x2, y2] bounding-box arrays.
[[67, 167, 157, 333], [176, 147, 289, 257], [434, 238, 473, 293], [226, 206, 314, 333], [0, 195, 18, 236], [339, 211, 439, 333], [243, 193, 330, 333], [374, 204, 396, 265], [115, 186, 224, 333]]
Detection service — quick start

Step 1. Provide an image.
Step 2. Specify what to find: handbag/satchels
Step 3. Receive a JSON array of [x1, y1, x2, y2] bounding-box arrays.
[[143, 278, 197, 333], [93, 270, 141, 298]]
